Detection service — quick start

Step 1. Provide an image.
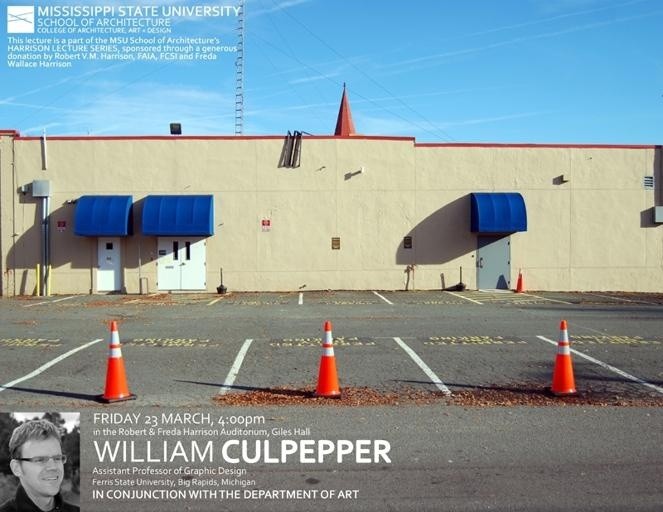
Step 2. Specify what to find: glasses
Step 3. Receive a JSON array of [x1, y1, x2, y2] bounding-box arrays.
[[12, 455, 67, 464]]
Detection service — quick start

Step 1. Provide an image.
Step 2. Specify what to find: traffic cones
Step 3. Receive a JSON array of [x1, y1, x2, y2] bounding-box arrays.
[[96, 320, 136, 403], [313, 321, 342, 399], [543, 320, 583, 398], [515, 268, 524, 292]]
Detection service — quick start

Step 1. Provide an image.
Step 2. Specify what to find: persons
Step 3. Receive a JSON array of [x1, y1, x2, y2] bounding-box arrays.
[[0, 420, 80, 512]]
